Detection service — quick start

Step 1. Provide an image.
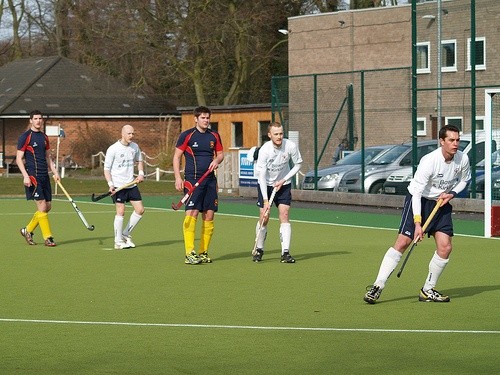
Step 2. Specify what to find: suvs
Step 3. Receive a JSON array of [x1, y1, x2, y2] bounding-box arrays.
[[337, 139, 440, 194], [301, 144, 402, 192], [384, 136, 496, 195]]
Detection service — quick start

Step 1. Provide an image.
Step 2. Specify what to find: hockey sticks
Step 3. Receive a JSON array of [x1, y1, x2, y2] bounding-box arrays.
[[397, 180, 460, 278], [172, 165, 214, 210], [252, 186, 278, 256], [53, 175, 95, 231], [92, 180, 138, 202]]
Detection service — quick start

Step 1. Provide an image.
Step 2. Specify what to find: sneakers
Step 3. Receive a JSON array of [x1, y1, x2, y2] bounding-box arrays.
[[253, 248, 263, 262], [363, 285, 382, 304], [122, 235, 135, 247], [281, 249, 295, 263], [45, 237, 55, 246], [419, 287, 450, 302], [115, 240, 130, 249], [185, 250, 202, 264], [21, 227, 35, 245], [199, 251, 212, 263]]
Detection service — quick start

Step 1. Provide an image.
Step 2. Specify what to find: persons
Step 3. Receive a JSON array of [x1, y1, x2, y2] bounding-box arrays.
[[173, 106, 224, 265], [252, 121, 304, 263], [363, 125, 472, 304], [16, 110, 61, 246], [333, 137, 358, 165], [103, 125, 145, 249]]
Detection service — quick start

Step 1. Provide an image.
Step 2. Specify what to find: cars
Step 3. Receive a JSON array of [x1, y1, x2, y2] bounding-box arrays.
[[455, 149, 500, 198], [468, 166, 500, 199]]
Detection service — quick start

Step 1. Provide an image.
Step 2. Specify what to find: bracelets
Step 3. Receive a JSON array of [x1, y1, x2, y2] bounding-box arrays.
[[264, 202, 269, 205], [414, 215, 422, 222], [138, 170, 144, 176]]
